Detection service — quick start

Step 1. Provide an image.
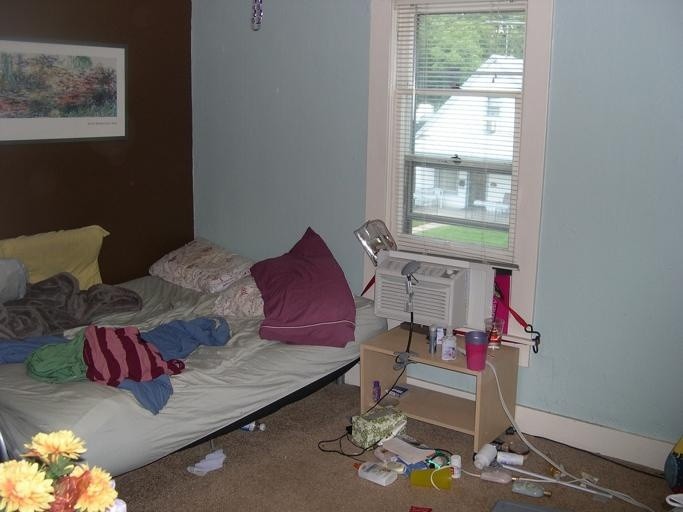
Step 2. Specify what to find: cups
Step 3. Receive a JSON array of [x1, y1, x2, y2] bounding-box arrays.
[[485, 317, 505, 351], [464, 332, 488, 370], [410, 468, 452, 491], [474, 443, 524, 469]]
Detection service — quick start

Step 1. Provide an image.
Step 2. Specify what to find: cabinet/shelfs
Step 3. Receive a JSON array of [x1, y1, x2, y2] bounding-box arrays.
[[360, 321, 519, 455]]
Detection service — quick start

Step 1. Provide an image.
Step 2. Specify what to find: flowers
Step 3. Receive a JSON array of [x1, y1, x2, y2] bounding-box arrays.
[[0, 430, 127, 512]]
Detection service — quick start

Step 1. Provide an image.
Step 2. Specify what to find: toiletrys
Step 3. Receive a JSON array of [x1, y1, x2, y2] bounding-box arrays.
[[480, 465, 520, 484], [352, 461, 397, 486], [511, 479, 552, 497], [440, 327, 457, 361], [428, 324, 436, 354]]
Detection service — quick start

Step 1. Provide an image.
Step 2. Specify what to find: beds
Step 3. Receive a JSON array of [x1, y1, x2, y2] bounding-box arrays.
[[0, 274, 388, 486]]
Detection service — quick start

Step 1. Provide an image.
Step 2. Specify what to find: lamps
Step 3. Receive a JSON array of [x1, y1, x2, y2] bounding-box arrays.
[[393, 260, 426, 370]]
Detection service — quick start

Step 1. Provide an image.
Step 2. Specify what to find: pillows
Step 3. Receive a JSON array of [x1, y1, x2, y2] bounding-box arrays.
[[213, 275, 263, 318], [147, 234, 255, 291], [1, 256, 28, 302], [0, 224, 108, 292], [252, 226, 356, 346]]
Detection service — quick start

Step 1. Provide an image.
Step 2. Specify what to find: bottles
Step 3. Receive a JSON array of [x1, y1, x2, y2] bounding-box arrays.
[[451, 455, 462, 478], [243, 422, 266, 432]]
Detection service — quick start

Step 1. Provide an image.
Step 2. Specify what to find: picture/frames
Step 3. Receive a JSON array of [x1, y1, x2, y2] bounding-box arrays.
[[0, 34, 129, 144]]
